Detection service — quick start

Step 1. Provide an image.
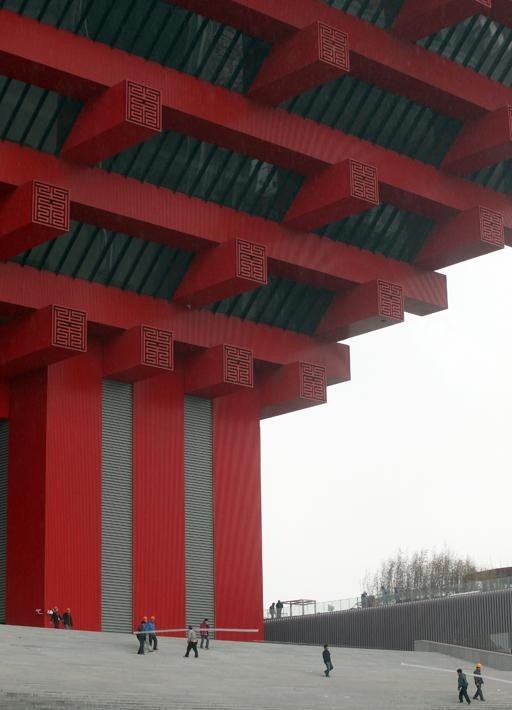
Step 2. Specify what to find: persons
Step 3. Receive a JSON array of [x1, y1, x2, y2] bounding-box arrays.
[[149, 617, 159, 650], [457, 668, 471, 705], [380, 584, 389, 605], [184, 625, 199, 657], [472, 663, 485, 701], [361, 592, 368, 609], [322, 643, 334, 677], [143, 616, 154, 653], [276, 600, 283, 618], [269, 602, 277, 619], [49, 607, 61, 628], [62, 607, 73, 629], [199, 617, 212, 649], [393, 586, 403, 604], [137, 621, 147, 654]]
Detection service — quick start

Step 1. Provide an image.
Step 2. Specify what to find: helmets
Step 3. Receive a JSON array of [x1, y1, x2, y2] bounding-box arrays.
[[476, 664, 481, 667]]
